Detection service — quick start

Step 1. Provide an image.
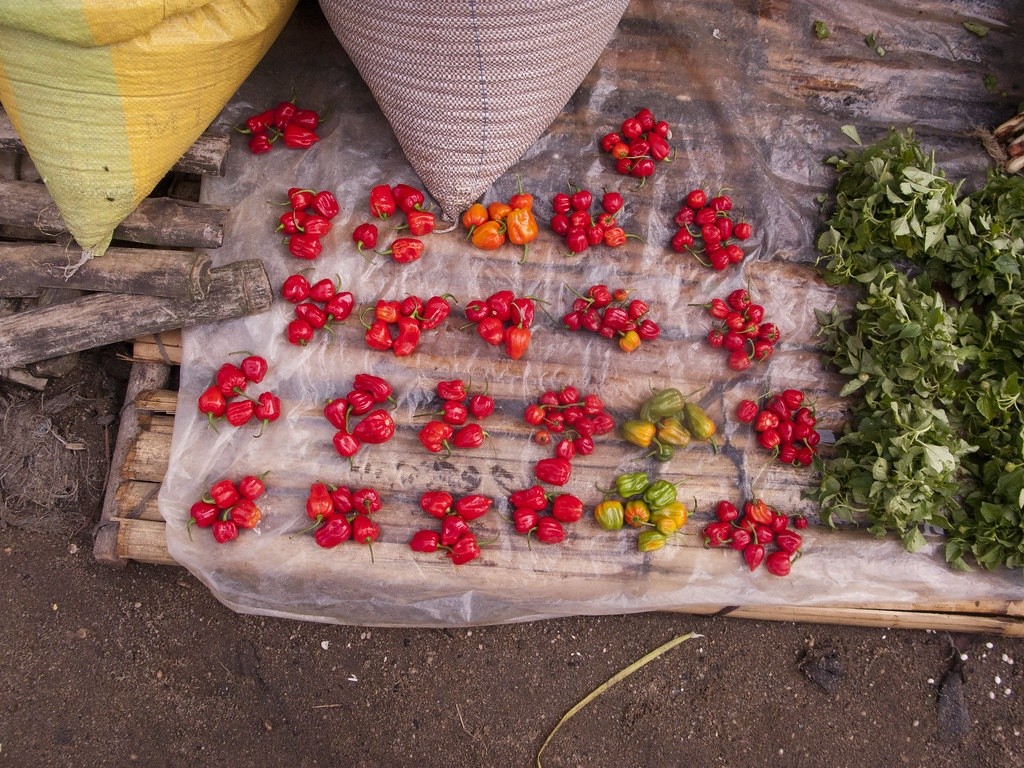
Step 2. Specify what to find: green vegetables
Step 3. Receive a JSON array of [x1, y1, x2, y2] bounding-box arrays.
[[802, 121, 1024, 574]]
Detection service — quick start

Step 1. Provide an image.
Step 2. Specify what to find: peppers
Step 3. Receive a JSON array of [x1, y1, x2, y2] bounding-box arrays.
[[184, 96, 821, 575]]
